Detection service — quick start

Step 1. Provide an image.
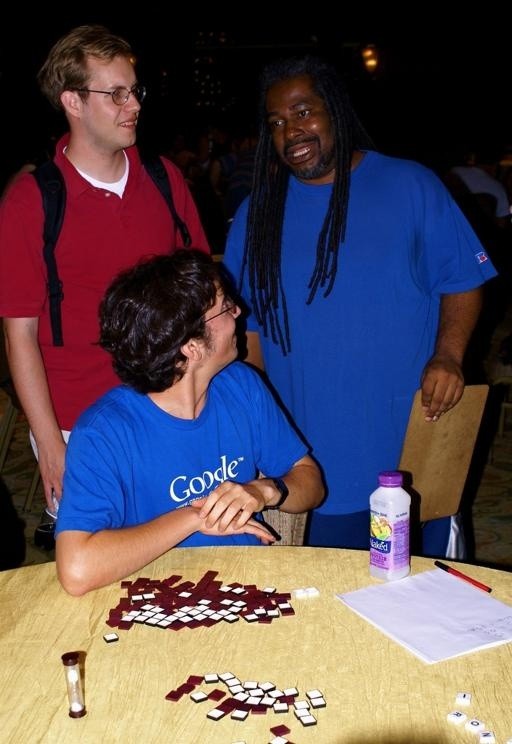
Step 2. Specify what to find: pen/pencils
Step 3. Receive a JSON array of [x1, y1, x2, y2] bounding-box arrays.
[[434, 561, 492, 593]]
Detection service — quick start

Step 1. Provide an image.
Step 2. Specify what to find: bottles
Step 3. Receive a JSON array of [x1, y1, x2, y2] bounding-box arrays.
[[367, 470, 413, 582]]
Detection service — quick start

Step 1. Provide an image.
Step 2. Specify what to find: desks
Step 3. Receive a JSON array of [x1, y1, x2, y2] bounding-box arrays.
[[0, 545, 511, 743]]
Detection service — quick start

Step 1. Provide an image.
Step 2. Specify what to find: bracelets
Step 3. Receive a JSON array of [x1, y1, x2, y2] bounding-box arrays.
[[259, 478, 288, 511]]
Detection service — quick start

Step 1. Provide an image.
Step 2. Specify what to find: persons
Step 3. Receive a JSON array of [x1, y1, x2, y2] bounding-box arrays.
[[50, 242, 329, 597], [219, 53, 501, 563], [0, 19, 214, 517], [442, 149, 510, 219]]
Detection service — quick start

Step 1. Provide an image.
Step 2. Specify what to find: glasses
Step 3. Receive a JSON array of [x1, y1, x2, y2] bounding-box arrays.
[[70, 85, 146, 105], [204, 295, 236, 322]]
